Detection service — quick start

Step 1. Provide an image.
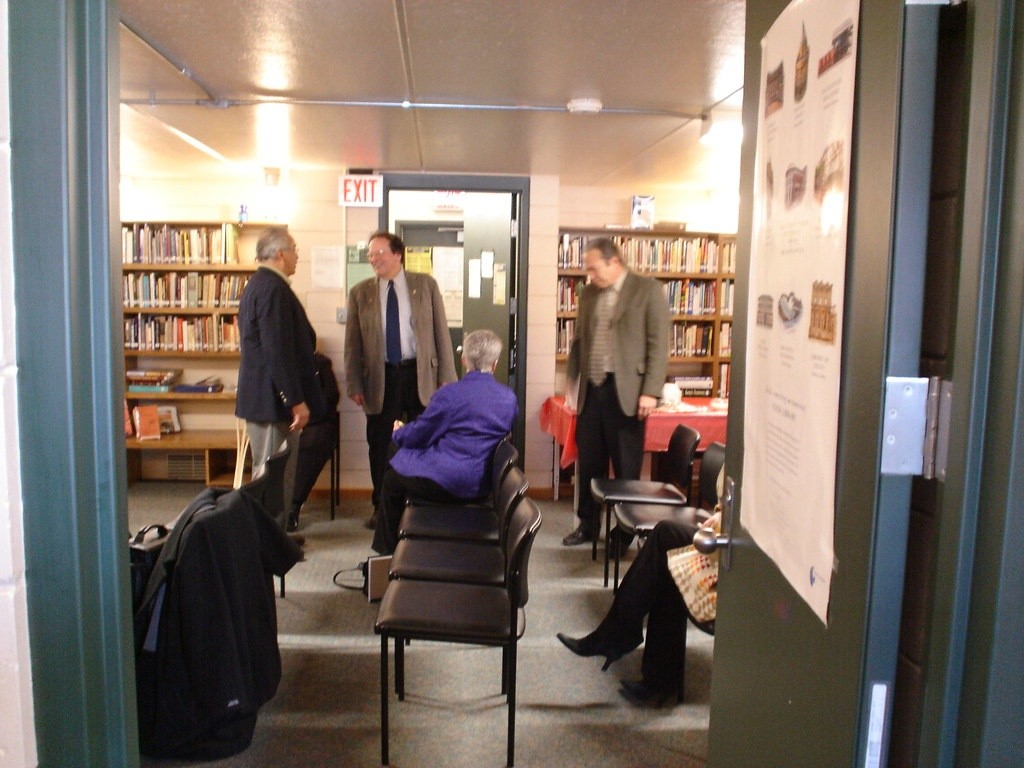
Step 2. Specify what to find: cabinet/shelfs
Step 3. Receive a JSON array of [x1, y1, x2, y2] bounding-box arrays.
[[557, 226, 737, 399], [121, 220, 288, 484]]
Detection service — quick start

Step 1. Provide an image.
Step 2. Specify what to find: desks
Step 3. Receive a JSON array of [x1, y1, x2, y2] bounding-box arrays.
[[540, 398, 728, 532]]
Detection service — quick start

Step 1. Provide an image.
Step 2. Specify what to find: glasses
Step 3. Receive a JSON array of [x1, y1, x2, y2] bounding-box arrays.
[[367, 248, 399, 259]]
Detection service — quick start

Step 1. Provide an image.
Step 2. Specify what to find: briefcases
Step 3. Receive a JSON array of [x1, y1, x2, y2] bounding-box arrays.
[[129, 521, 172, 614]]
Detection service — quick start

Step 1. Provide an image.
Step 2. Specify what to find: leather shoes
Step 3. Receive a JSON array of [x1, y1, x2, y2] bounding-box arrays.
[[563, 527, 595, 545], [609, 537, 629, 559], [368, 509, 381, 529]]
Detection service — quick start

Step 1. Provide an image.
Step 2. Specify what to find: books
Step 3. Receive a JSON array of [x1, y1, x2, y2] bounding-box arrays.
[[720, 323, 732, 357], [559, 275, 591, 313], [123, 271, 253, 309], [556, 318, 576, 355], [671, 322, 713, 356], [559, 234, 590, 271], [719, 364, 730, 397], [662, 278, 716, 316], [125, 400, 180, 440], [127, 367, 223, 393], [124, 312, 241, 352], [669, 375, 713, 396], [122, 223, 240, 264], [722, 277, 734, 315], [610, 236, 737, 274]]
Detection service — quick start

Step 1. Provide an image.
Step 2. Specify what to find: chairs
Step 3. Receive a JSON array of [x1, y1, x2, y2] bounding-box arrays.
[[374, 431, 543, 768], [328, 413, 340, 520], [612, 441, 726, 592], [590, 423, 701, 588]]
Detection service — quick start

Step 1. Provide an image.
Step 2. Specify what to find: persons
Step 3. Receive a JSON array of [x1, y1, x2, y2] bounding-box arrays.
[[370, 331, 519, 556], [344, 232, 458, 527], [556, 463, 724, 706], [235, 229, 316, 562], [563, 238, 669, 557]]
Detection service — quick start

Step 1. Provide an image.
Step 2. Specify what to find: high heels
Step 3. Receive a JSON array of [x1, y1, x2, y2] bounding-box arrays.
[[619, 676, 672, 709], [558, 619, 625, 671]]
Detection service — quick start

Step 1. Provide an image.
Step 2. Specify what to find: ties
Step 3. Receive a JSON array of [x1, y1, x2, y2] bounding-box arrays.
[[587, 291, 613, 388], [385, 280, 402, 367]]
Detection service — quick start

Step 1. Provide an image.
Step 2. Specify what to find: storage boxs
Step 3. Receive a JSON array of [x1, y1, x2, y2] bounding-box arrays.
[[140, 450, 205, 478]]
